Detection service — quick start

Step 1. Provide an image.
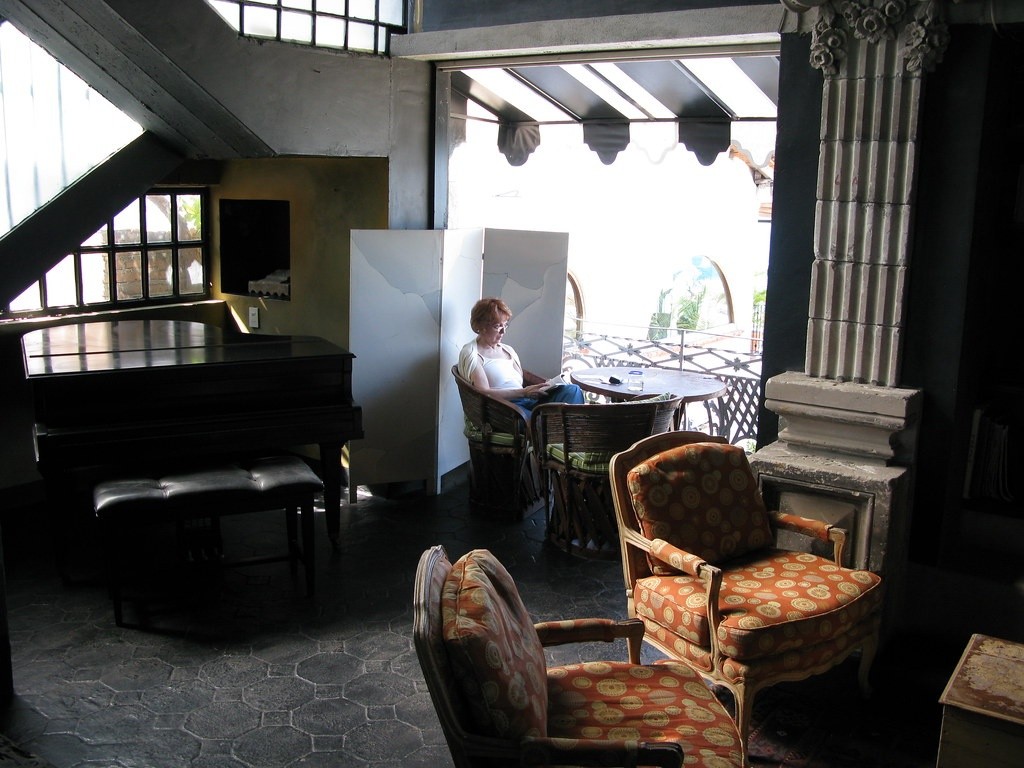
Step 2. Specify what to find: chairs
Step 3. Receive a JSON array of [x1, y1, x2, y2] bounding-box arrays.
[[451, 361, 578, 523], [609, 429, 886, 762], [530, 393, 686, 561], [409, 543, 749, 767]]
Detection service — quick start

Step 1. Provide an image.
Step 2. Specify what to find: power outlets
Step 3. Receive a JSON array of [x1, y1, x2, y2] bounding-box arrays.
[[248, 307, 259, 328]]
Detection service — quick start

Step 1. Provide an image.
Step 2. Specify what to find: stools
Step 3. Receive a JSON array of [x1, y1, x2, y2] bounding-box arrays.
[[935, 633, 1024, 768]]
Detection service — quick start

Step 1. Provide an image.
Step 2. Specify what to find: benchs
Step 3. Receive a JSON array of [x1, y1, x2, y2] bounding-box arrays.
[[93, 449, 326, 628]]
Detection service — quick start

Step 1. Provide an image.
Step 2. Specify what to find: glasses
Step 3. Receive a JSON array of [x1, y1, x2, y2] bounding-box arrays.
[[487, 322, 509, 332]]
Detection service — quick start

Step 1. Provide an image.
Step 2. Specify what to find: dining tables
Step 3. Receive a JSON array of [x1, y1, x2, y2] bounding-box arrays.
[[570, 367, 727, 433]]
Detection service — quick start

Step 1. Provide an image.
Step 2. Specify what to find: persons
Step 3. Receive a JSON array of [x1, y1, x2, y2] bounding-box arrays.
[[457, 298, 583, 429]]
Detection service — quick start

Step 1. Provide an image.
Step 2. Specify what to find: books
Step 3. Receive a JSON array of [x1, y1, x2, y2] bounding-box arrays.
[[540, 374, 568, 392]]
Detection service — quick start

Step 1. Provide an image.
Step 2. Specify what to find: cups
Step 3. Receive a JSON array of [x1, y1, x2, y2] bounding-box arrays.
[[627, 371, 643, 391]]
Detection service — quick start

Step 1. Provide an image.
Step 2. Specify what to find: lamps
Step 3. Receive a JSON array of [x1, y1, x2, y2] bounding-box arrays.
[[497, 120, 541, 166], [676, 116, 732, 166], [582, 118, 630, 165]]
